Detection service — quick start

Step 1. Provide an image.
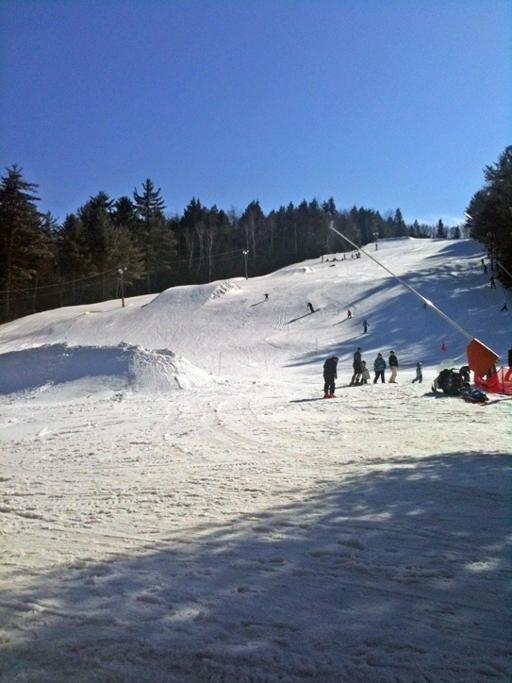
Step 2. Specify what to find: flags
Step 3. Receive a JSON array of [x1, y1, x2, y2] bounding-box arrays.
[[439, 341, 448, 351]]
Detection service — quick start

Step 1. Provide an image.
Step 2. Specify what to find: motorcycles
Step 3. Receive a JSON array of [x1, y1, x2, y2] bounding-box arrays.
[[459, 385, 488, 403]]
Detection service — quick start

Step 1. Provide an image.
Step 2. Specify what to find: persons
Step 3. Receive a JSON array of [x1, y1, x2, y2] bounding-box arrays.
[[372, 351, 386, 383], [306, 301, 316, 312], [483, 265, 488, 273], [488, 272, 497, 289], [360, 358, 370, 384], [387, 348, 399, 383], [355, 251, 358, 258], [350, 346, 362, 385], [347, 308, 352, 319], [263, 292, 270, 301], [458, 362, 471, 381], [362, 318, 370, 332], [411, 359, 422, 383], [480, 257, 484, 269], [322, 354, 340, 397]]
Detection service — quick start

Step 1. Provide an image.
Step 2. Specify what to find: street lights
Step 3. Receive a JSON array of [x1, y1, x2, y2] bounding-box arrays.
[[373, 232, 380, 250], [118, 266, 127, 307], [486, 231, 496, 271], [243, 250, 250, 280]]
